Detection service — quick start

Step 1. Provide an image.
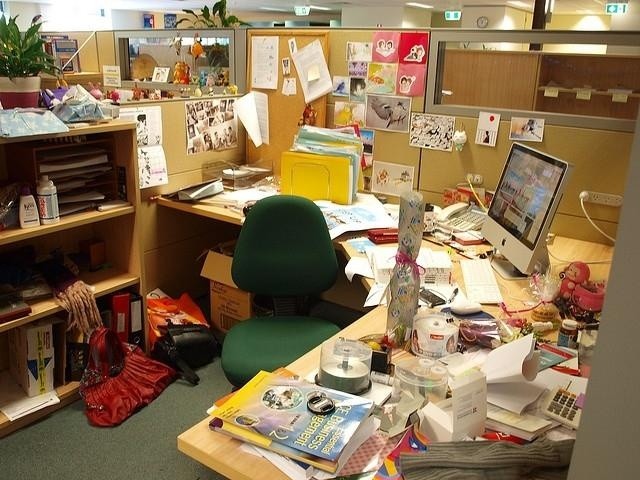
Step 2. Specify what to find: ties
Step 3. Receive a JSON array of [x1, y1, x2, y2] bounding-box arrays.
[[480, 142, 574, 281]]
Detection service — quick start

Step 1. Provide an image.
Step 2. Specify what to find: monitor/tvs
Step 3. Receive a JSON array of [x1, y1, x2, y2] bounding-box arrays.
[[452, 230, 484, 245], [206, 366, 381, 473], [485, 403, 551, 442]]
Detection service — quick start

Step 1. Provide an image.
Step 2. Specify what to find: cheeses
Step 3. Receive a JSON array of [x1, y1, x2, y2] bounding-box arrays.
[[320, 360, 369, 394]]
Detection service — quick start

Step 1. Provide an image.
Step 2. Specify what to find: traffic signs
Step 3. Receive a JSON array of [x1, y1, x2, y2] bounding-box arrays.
[[466, 173, 483, 186]]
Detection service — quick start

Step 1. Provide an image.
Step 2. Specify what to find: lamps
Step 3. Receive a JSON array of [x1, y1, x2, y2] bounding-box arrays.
[[218, 191, 347, 390]]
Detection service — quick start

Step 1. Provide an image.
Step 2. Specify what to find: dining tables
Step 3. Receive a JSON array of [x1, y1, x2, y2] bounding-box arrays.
[[0, 12, 63, 110], [174, 1, 253, 66]]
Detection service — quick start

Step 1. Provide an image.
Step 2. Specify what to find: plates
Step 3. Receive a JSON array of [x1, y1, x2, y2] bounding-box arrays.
[[0, 118, 141, 441]]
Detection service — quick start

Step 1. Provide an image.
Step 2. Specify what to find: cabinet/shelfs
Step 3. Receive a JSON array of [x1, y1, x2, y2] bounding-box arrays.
[[392, 356, 448, 416], [555, 319, 578, 348], [33, 174, 65, 226], [18, 184, 41, 230]]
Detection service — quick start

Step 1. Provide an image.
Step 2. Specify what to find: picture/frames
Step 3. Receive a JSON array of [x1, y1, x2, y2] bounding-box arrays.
[[154, 178, 616, 478]]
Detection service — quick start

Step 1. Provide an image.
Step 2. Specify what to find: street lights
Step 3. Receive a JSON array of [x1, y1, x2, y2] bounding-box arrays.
[[145, 291, 212, 351], [152, 318, 219, 386], [80, 326, 177, 431]]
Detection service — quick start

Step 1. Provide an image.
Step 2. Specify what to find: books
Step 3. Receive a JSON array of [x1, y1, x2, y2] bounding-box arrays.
[[195, 238, 275, 336], [5, 322, 54, 396]]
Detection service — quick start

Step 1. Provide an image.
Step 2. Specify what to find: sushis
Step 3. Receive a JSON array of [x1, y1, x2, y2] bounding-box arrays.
[[542, 386, 585, 433]]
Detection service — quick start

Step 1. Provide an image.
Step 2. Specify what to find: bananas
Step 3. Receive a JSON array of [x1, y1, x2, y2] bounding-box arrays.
[[460, 258, 503, 305]]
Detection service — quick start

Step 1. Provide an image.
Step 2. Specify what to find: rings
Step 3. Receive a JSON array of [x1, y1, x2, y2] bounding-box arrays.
[[448, 287, 458, 304]]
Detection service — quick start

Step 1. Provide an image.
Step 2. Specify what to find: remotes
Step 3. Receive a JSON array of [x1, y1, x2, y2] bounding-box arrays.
[[450, 300, 482, 315]]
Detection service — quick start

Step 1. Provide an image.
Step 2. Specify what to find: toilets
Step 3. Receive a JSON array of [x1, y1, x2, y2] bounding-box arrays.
[[432, 202, 487, 233]]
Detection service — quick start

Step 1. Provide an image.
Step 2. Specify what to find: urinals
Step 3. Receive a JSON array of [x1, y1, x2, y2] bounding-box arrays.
[[452, 233, 484, 245]]
